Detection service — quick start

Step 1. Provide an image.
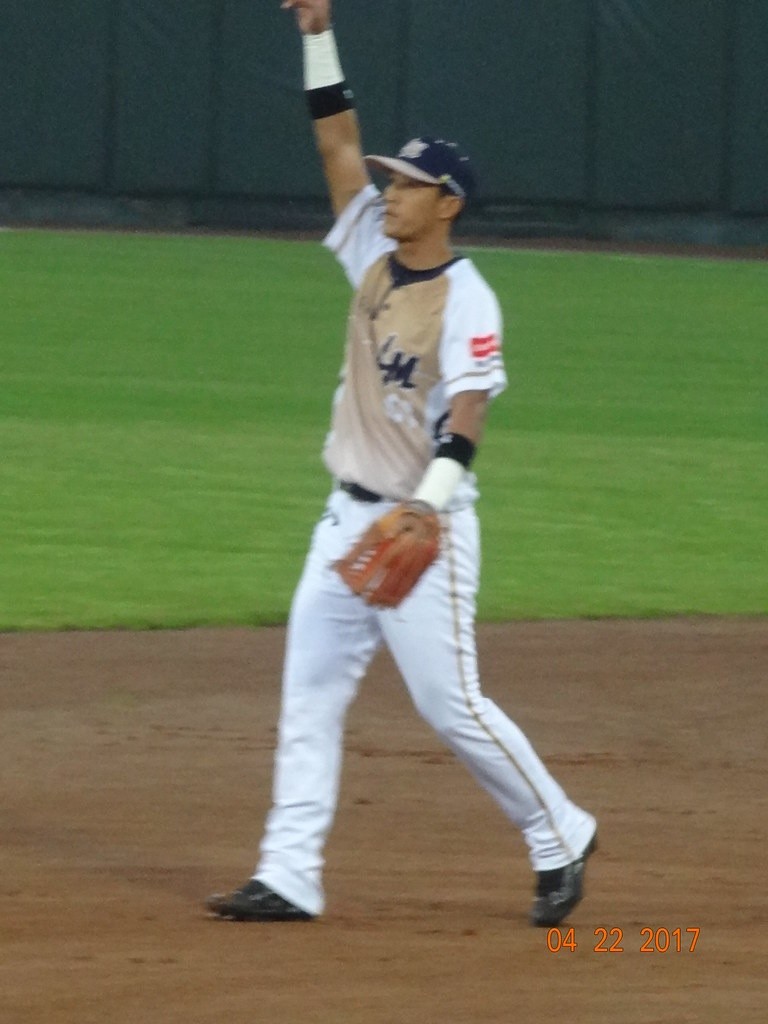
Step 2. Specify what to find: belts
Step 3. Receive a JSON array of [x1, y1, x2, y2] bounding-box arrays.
[[337, 482, 382, 503]]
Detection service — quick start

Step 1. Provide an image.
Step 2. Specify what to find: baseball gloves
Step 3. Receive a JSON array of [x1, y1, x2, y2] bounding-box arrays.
[[326, 498, 452, 612]]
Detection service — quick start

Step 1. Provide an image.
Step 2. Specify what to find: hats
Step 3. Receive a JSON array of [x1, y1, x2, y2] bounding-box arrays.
[[363, 136, 471, 204]]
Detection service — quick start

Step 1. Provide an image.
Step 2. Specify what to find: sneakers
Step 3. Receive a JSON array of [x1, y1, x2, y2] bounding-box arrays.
[[208, 879, 312, 921], [532, 827, 597, 926]]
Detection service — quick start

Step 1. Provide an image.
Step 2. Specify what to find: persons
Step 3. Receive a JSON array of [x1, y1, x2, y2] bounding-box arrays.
[[201, 0, 602, 925]]
